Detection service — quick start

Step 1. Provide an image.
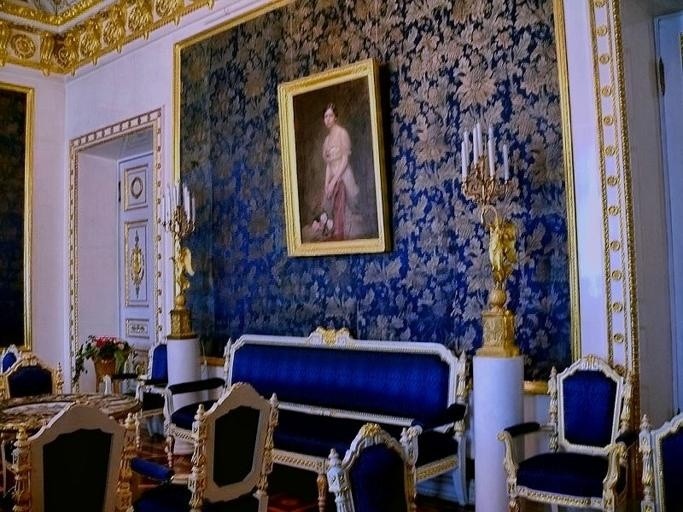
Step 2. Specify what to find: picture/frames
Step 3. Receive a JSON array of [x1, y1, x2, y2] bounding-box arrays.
[[277, 58, 391, 259], [0, 81, 34, 354]]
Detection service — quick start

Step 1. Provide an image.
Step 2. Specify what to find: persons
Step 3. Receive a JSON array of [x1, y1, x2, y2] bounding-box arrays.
[[319, 102, 363, 241]]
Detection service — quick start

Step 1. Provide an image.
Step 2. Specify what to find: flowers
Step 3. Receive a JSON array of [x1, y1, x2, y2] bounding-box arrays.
[[71, 335, 133, 386]]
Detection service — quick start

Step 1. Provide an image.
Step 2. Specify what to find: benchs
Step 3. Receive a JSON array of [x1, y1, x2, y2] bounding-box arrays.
[[162, 320, 469, 512]]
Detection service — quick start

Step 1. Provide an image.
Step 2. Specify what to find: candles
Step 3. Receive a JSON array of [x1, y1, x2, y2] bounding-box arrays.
[[162, 182, 196, 224], [461, 121, 510, 183]]
